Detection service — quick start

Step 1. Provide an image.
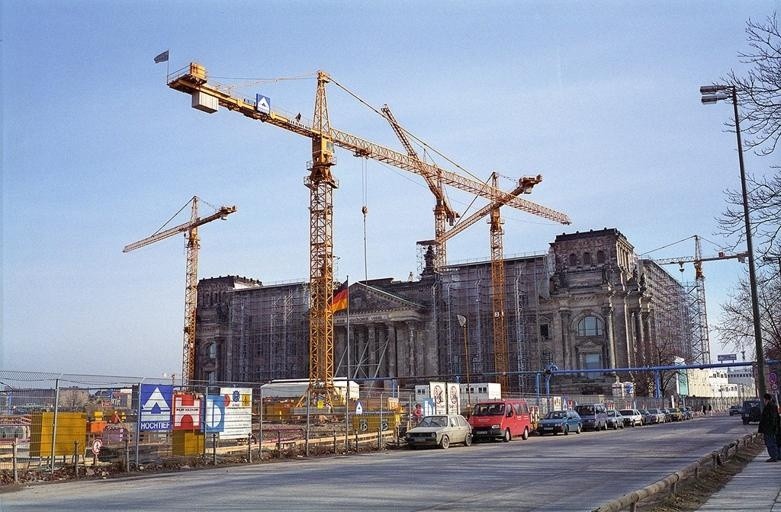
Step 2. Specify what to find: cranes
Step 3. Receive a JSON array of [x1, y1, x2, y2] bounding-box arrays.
[[653, 235, 749, 366], [123, 195, 239, 394]]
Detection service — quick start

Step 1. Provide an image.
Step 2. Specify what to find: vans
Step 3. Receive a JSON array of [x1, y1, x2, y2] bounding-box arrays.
[[468, 401, 532, 442], [575, 404, 609, 431]]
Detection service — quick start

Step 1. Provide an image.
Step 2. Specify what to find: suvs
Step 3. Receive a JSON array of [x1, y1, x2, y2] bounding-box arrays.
[[730, 401, 761, 424]]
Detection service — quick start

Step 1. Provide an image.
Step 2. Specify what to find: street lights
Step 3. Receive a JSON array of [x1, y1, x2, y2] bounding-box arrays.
[[699, 84, 765, 413]]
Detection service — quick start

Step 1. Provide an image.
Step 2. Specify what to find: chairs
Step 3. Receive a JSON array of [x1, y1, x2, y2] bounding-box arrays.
[[482, 406, 505, 413]]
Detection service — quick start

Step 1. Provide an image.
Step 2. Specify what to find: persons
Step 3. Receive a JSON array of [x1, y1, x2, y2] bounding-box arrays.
[[703, 403, 706, 416], [772, 398, 781, 460], [412, 403, 424, 427], [757, 393, 778, 463], [708, 404, 712, 416]]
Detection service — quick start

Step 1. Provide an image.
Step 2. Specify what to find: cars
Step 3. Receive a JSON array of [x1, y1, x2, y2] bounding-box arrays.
[[404, 414, 474, 448], [606, 407, 693, 430], [537, 410, 582, 434]]
